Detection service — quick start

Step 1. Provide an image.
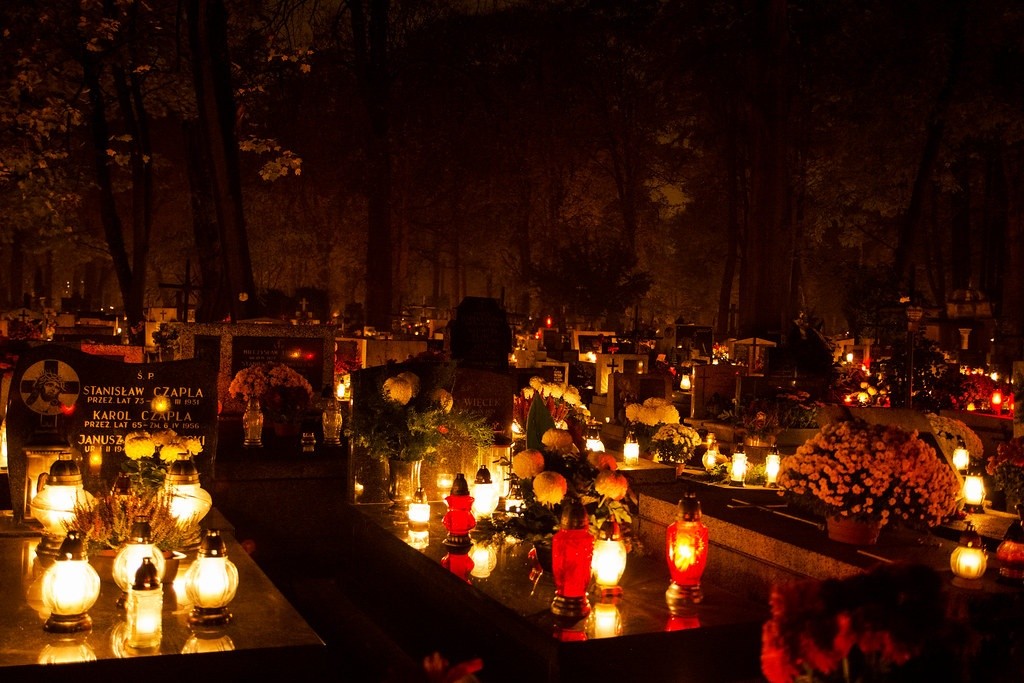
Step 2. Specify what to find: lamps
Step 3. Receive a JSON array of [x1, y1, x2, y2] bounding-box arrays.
[[186, 528, 240, 625], [592, 512, 627, 595], [950, 460, 1024, 590], [665, 493, 709, 599], [678, 370, 692, 392], [38, 529, 101, 632], [730, 443, 748, 487], [157, 450, 212, 547], [764, 447, 782, 487], [32, 452, 97, 555], [406, 488, 430, 526], [442, 473, 473, 548], [622, 427, 639, 465], [584, 431, 605, 452], [551, 494, 591, 617], [112, 513, 166, 609], [700, 444, 719, 471], [472, 465, 498, 514], [952, 441, 970, 476], [126, 557, 165, 647]]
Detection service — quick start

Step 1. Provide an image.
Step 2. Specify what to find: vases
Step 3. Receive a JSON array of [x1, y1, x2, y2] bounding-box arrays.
[[98, 551, 185, 587], [242, 399, 265, 447], [272, 418, 303, 436], [320, 399, 344, 445], [161, 349, 175, 362], [535, 352, 548, 362], [743, 435, 774, 464], [386, 459, 424, 505], [687, 372, 692, 385], [823, 507, 884, 545], [533, 543, 553, 576], [778, 428, 821, 447], [659, 461, 686, 477]]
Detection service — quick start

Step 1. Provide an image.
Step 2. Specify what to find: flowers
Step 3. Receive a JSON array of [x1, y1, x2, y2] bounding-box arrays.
[[470, 394, 646, 559], [651, 423, 702, 463], [522, 376, 582, 421], [339, 354, 498, 475], [718, 396, 780, 444], [778, 391, 825, 428], [985, 436, 1024, 503], [268, 362, 313, 415], [681, 361, 700, 371], [537, 340, 546, 351], [229, 362, 274, 401], [65, 432, 205, 553], [776, 421, 960, 529], [152, 322, 181, 353], [926, 412, 984, 461]]
